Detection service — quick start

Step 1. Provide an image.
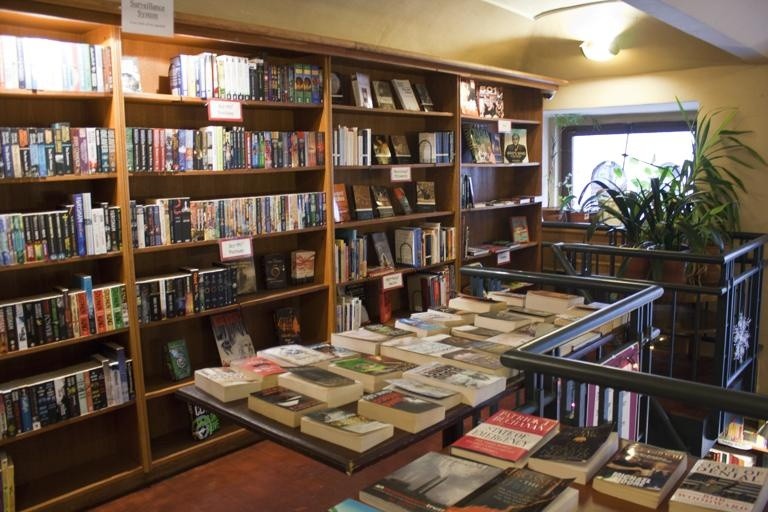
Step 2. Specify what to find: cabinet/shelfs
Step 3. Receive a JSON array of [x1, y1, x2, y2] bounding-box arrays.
[[460, 61, 569, 295], [330, 39, 461, 336], [0, 9, 151, 512], [116, 24, 330, 483]]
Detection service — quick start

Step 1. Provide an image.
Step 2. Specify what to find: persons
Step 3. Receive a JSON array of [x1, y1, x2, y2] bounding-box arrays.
[[638, 460, 671, 489], [692, 474, 745, 496], [596, 453, 644, 483], [557, 435, 587, 462]]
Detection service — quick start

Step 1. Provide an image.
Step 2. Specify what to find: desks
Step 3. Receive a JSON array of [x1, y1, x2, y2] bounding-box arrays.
[[176, 279, 636, 478], [647, 290, 721, 386], [325, 417, 699, 512]]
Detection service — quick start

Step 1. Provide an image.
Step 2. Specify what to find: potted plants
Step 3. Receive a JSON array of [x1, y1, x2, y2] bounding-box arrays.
[[579, 95, 766, 301]]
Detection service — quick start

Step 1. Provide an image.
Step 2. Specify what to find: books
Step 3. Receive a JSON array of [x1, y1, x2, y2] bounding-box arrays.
[[447, 409, 561, 468], [360, 450, 507, 511], [669, 457, 768, 512], [0, 452, 16, 512], [329, 498, 381, 511], [708, 415, 768, 467], [591, 441, 689, 509], [445, 467, 580, 511], [527, 423, 621, 486]]
